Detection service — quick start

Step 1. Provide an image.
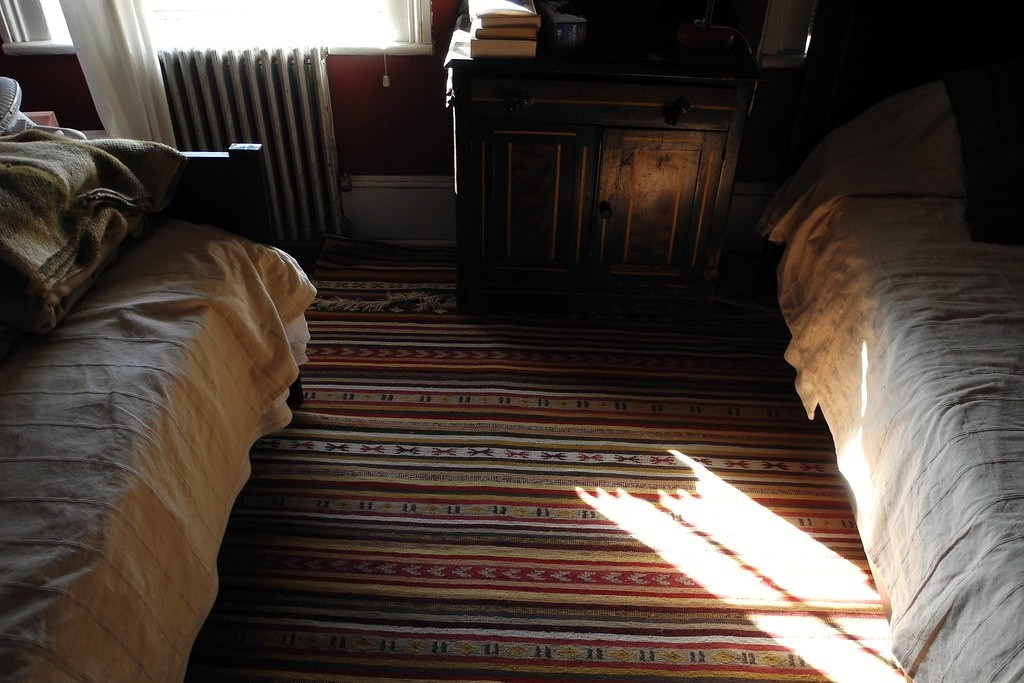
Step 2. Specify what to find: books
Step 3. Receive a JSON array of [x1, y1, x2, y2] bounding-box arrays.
[[469, 0, 542, 56]]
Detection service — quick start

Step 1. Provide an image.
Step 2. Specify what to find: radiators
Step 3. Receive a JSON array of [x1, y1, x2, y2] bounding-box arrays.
[[156, 46, 347, 249]]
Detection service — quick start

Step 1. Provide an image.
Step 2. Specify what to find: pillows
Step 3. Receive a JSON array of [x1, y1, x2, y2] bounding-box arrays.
[[940, 59, 1024, 245]]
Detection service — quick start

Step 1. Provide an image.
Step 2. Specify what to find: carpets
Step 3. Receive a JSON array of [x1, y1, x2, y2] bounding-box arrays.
[[184, 235, 873, 683]]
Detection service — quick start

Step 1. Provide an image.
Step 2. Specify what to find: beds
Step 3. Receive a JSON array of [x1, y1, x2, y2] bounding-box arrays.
[[765, 1, 1024, 683], [1, 145, 319, 682]]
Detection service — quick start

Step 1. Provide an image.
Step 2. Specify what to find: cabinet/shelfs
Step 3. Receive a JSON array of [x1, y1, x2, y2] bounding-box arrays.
[[445, 15, 761, 314]]
